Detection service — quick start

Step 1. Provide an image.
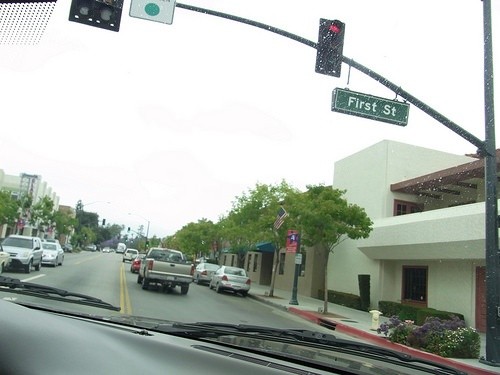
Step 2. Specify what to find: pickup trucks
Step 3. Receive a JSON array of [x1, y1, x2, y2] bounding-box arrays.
[[137, 247, 195, 295]]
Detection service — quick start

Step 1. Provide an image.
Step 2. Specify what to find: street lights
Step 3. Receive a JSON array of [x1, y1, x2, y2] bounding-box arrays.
[[288, 214, 304, 305], [76, 201, 111, 219], [128, 212, 151, 237]]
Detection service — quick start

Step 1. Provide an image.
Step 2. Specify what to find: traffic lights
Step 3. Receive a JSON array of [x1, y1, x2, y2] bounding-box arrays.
[[67, 0, 124, 32], [314, 18, 347, 77], [146, 241, 149, 247]]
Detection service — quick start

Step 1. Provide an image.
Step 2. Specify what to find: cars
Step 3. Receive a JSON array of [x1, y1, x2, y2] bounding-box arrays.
[[0, 242, 10, 273], [103, 247, 114, 253], [41, 242, 64, 267], [130, 254, 145, 273], [63, 244, 73, 253], [210, 266, 251, 296], [85, 244, 97, 251], [193, 262, 220, 285]]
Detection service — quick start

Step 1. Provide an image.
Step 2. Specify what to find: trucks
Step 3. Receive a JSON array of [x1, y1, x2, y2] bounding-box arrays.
[[116, 243, 126, 253]]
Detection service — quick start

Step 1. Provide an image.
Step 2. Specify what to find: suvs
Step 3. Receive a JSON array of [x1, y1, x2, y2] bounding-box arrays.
[[0, 234, 43, 273], [123, 248, 139, 262]]
